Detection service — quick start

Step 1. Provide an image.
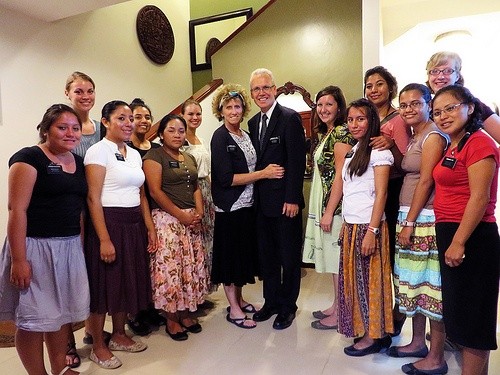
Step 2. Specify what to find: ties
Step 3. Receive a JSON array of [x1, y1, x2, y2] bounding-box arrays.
[[259, 114, 268, 152]]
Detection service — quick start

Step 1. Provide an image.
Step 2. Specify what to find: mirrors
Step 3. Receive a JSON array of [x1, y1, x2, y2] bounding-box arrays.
[[275, 81, 317, 178], [189, 8, 253, 72]]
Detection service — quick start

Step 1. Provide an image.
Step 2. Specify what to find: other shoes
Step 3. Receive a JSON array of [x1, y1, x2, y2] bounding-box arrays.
[[354, 333, 393, 348], [187, 299, 213, 318], [311, 318, 338, 330], [89, 347, 122, 369], [108, 339, 147, 353], [127, 312, 167, 337], [344, 342, 381, 357], [180, 320, 202, 333], [402, 359, 448, 375], [386, 346, 429, 359], [313, 310, 331, 319], [165, 324, 188, 341]]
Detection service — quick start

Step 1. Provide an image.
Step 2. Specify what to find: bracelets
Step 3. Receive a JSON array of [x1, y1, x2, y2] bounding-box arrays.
[[402, 219, 416, 228]]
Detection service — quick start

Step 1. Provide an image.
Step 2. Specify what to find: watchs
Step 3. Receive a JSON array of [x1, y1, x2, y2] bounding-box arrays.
[[367, 226, 379, 234]]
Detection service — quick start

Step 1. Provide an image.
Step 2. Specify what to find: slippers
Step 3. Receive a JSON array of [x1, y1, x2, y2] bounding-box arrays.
[[226, 312, 256, 329], [227, 303, 256, 313]]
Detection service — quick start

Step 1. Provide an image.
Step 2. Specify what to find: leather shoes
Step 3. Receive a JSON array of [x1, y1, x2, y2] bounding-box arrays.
[[253, 299, 276, 322], [272, 310, 296, 329]]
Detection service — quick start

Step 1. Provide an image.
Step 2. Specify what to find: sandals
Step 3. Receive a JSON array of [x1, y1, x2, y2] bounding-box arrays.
[[64, 342, 81, 368], [82, 328, 112, 344]]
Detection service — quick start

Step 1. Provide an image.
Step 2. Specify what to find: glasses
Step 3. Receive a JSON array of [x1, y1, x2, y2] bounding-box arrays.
[[430, 102, 464, 118], [397, 101, 422, 110], [252, 84, 274, 92], [429, 68, 456, 76]]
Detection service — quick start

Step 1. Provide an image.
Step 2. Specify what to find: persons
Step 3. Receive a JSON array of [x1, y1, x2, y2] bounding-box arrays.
[[431, 85, 500, 375], [122, 97, 161, 158], [302, 85, 359, 330], [178, 98, 215, 246], [209, 82, 285, 330], [385, 82, 452, 375], [142, 113, 210, 339], [246, 68, 306, 329], [426, 51, 500, 351], [337, 98, 394, 357], [0, 104, 90, 375], [363, 66, 407, 336], [65, 72, 112, 368], [84, 100, 159, 368]]
